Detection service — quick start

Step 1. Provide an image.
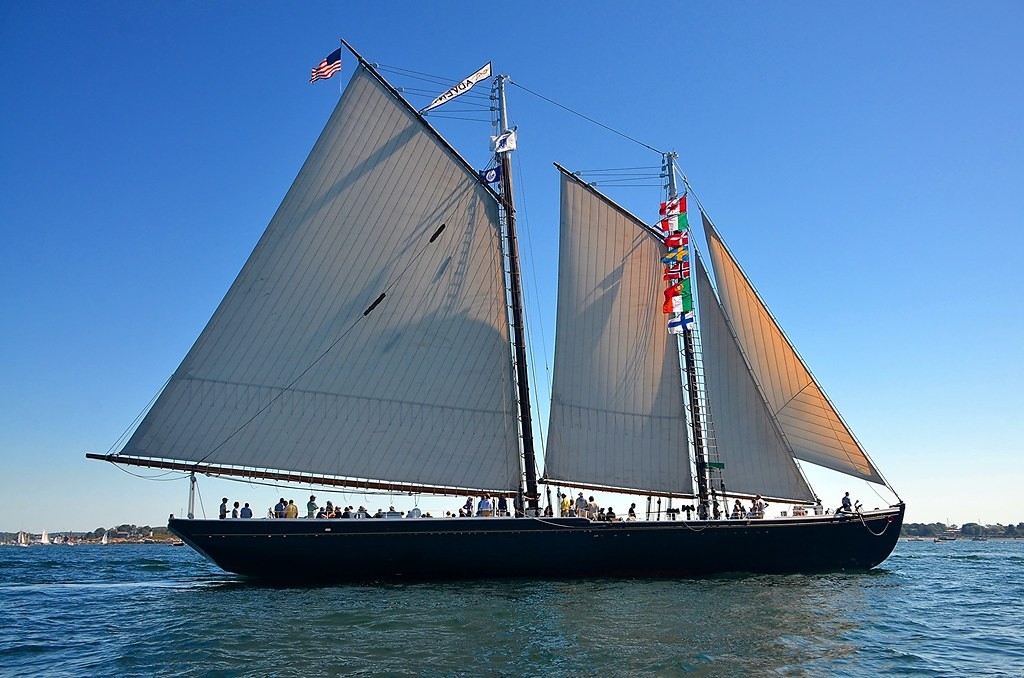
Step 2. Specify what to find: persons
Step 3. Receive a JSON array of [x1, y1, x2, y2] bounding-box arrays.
[[232, 502, 239, 518], [219, 498, 230, 519], [274, 492, 636, 521], [696, 492, 852, 520], [241, 503, 252, 517]]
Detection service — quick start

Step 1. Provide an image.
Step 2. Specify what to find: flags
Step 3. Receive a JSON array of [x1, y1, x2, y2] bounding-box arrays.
[[309, 47, 342, 85], [659, 196, 695, 334], [479, 166, 501, 183]]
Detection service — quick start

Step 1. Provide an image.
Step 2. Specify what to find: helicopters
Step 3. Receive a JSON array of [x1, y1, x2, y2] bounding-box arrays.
[[83, 38, 907, 584]]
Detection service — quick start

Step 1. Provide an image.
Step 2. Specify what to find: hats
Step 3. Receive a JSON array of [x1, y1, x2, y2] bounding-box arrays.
[[222, 497, 229, 500], [389, 506, 395, 510], [445, 511, 451, 515], [578, 492, 583, 496], [310, 495, 317, 498], [486, 493, 491, 498]]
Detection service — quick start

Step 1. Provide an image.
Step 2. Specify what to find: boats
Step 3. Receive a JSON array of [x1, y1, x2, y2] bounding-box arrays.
[[911, 538, 992, 543]]
